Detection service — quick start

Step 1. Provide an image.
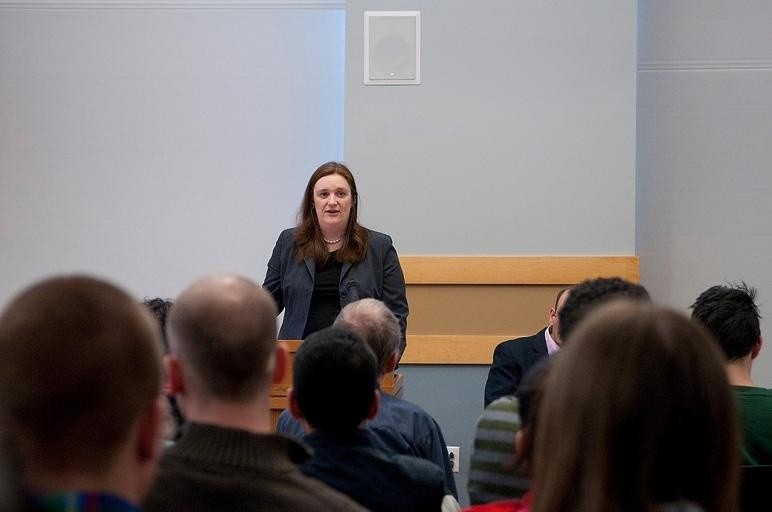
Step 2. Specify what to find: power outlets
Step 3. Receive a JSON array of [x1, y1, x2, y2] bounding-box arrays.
[[446, 446, 460, 473]]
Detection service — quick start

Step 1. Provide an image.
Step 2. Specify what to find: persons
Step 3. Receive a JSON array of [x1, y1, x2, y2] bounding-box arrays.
[[286, 326, 451, 512], [275, 296, 461, 500], [137, 296, 187, 443], [525, 294, 738, 511], [262, 160, 410, 371], [0, 274, 173, 512], [459, 345, 566, 512], [686, 278, 771, 469], [482, 286, 576, 412], [137, 270, 374, 512], [466, 274, 654, 507]]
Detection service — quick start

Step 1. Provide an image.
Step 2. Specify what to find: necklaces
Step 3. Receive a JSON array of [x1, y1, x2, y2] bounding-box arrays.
[[322, 236, 344, 245]]
